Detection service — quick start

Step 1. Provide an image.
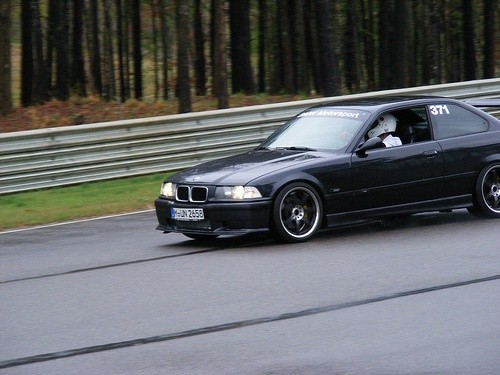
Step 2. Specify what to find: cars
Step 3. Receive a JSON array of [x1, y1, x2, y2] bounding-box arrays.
[[152, 94, 500, 243]]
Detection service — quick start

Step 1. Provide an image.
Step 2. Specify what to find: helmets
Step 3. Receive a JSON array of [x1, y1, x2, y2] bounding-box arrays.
[[368, 113, 397, 140]]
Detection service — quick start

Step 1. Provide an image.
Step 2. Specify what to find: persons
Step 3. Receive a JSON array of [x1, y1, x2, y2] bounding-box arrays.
[[364, 115, 403, 148]]
